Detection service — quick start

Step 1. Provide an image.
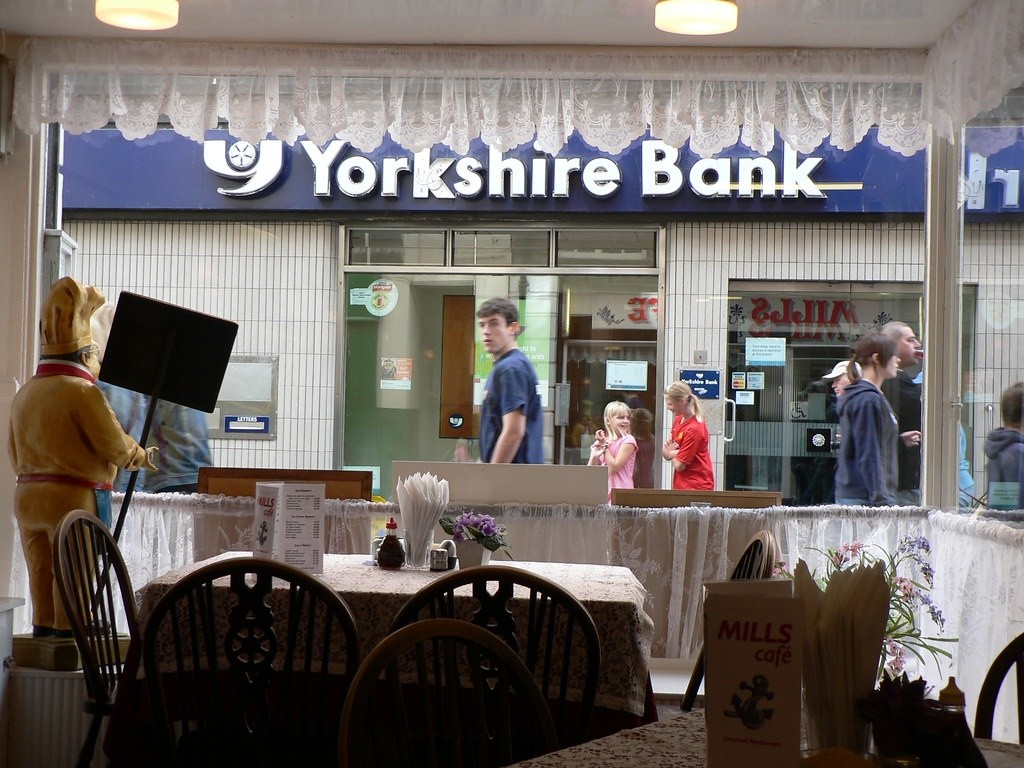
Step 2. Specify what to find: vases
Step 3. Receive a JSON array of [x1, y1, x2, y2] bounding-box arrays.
[[454, 540, 491, 570]]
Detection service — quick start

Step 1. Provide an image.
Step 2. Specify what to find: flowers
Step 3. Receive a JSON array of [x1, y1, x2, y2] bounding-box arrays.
[[771, 537, 959, 681], [438, 509, 512, 561]]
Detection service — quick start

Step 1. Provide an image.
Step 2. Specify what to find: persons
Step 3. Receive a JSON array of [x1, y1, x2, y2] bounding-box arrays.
[[89, 302, 213, 494], [792, 369, 839, 506], [624, 393, 656, 489], [822, 320, 1023, 511], [570, 399, 599, 448], [587, 400, 640, 507], [661, 381, 714, 491], [477, 297, 544, 464], [6, 275, 159, 638], [381, 358, 395, 380]]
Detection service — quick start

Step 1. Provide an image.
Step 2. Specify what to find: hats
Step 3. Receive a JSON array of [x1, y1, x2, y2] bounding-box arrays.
[[821, 361, 863, 379]]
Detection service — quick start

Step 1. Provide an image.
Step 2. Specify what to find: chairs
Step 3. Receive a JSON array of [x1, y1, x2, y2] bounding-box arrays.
[[52, 509, 777, 768], [974, 633, 1024, 745]]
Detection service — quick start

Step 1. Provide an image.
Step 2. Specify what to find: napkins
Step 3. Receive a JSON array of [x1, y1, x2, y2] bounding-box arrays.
[[794, 558, 889, 756], [396, 471, 450, 567]]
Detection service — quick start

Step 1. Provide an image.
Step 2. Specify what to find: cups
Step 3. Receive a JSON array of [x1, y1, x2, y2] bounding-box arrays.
[[403, 529, 434, 570]]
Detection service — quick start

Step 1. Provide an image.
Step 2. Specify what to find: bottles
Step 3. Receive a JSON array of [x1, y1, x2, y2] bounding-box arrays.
[[920, 678, 971, 768], [377, 517, 406, 570]]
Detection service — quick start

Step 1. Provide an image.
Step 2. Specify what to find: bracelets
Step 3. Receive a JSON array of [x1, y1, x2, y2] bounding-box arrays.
[[600, 441, 610, 449]]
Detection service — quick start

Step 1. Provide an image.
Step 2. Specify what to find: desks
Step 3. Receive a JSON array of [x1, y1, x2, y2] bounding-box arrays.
[[103, 551, 659, 768], [509, 708, 1024, 767]]
